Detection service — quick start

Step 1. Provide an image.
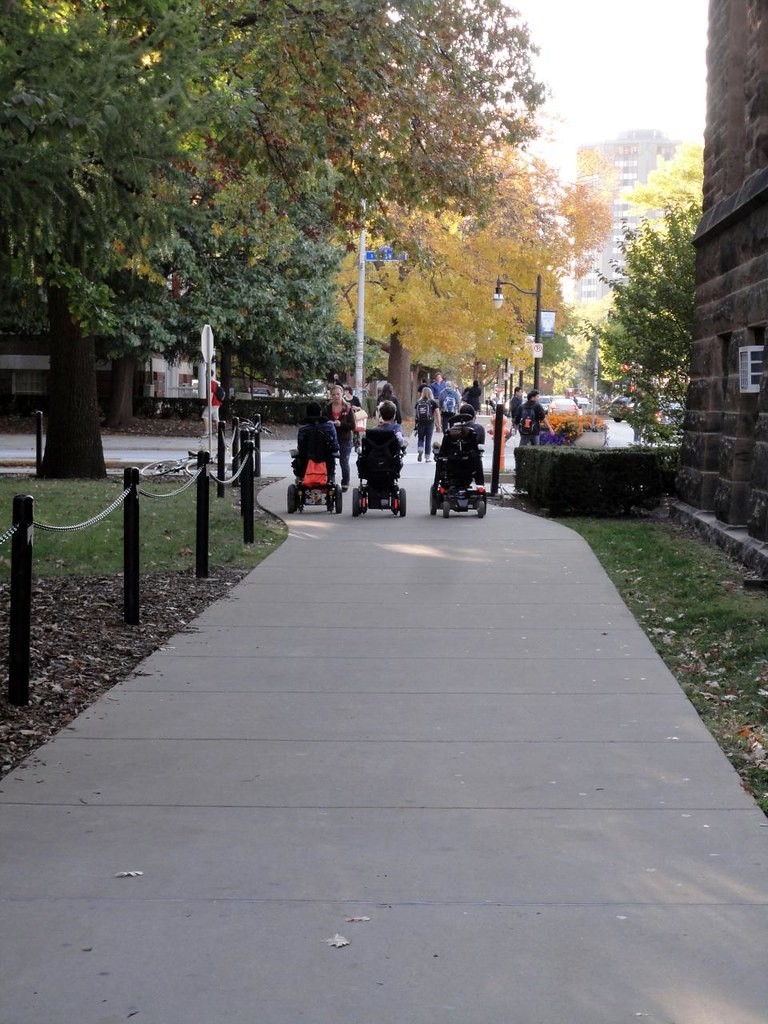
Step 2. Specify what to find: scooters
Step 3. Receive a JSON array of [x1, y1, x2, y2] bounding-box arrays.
[[429, 413, 487, 518], [352, 428, 406, 518], [287, 416, 343, 514]]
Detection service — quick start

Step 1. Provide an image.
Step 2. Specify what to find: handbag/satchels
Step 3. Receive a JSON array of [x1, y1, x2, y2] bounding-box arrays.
[[353, 406, 368, 432], [302, 459, 328, 488]]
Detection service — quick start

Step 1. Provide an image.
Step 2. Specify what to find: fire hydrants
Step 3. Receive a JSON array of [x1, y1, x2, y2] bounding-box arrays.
[[486, 413, 513, 473]]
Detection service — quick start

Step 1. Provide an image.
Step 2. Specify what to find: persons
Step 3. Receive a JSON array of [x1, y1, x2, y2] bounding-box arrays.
[[377, 383, 402, 425], [321, 385, 356, 493], [413, 387, 442, 463], [509, 387, 523, 431], [514, 390, 555, 446], [439, 381, 460, 434], [373, 400, 409, 469], [462, 387, 471, 402], [297, 402, 339, 452], [200, 370, 222, 438], [431, 372, 446, 432], [418, 378, 431, 396], [487, 397, 496, 412], [344, 385, 361, 452], [468, 380, 482, 422], [453, 404, 484, 486]]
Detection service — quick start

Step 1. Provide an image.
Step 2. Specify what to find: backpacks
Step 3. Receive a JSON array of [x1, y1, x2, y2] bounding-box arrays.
[[415, 400, 434, 425], [519, 404, 540, 435], [212, 379, 225, 401], [439, 390, 459, 416]]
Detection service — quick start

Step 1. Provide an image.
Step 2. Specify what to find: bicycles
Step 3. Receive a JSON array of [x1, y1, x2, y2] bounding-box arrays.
[[139, 450, 228, 478]]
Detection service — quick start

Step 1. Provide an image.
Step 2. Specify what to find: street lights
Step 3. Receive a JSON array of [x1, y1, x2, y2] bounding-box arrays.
[[592, 323, 602, 413], [492, 281, 540, 400]]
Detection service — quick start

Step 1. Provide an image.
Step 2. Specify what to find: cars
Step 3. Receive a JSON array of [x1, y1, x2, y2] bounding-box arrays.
[[574, 396, 590, 409], [550, 396, 564, 401], [548, 399, 578, 416], [535, 396, 552, 410], [609, 396, 639, 422], [253, 387, 271, 400], [283, 379, 329, 397], [565, 387, 578, 398]]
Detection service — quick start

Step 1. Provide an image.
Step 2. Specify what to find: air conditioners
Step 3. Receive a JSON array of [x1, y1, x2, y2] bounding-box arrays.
[[739, 345, 765, 393]]
